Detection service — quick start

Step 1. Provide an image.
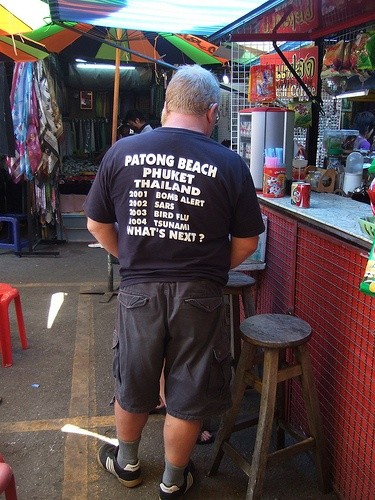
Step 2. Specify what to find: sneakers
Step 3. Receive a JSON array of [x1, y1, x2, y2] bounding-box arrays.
[[159, 458, 196, 500], [97, 442, 143, 488]]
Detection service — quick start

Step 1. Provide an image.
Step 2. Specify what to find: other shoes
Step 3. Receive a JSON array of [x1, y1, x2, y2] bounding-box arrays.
[[148, 399, 167, 416], [196, 426, 214, 445]]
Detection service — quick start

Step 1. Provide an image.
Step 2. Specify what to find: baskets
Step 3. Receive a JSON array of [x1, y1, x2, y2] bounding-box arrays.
[[358, 216, 375, 242]]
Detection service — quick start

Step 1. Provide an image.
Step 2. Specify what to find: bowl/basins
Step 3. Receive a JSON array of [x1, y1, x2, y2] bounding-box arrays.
[[358, 217, 375, 239]]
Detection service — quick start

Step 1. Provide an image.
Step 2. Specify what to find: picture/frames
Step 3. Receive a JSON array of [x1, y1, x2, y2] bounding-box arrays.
[[79, 90, 93, 110]]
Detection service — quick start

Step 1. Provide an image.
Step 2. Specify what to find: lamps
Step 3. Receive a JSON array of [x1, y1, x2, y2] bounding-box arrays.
[[332, 89, 368, 99]]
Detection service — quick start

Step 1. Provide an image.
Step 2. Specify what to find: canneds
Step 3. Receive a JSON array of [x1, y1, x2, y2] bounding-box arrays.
[[291, 182, 311, 209]]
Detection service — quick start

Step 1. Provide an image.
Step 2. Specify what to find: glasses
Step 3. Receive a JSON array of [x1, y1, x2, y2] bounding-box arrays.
[[208, 102, 222, 126]]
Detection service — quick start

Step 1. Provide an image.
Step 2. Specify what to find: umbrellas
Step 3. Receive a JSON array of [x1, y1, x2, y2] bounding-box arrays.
[[0, 0, 286, 291]]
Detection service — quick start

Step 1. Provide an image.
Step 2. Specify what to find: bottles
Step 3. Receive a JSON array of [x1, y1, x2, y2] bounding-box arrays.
[[307, 171, 321, 187], [342, 148, 363, 194], [326, 148, 343, 172], [255, 98, 259, 108]]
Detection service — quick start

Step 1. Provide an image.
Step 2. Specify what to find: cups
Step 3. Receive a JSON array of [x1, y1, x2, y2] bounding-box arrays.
[[280, 96, 289, 109], [263, 168, 286, 198]]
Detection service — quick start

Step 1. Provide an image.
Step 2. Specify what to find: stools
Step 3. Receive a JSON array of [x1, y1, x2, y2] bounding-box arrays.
[[0, 282, 29, 368], [206, 313, 327, 500], [225, 272, 256, 390], [0, 213, 32, 257]]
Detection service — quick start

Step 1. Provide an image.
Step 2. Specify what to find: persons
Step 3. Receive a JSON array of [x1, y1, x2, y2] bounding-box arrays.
[[82, 64, 266, 500], [348, 111, 375, 150], [125, 109, 153, 134]]
